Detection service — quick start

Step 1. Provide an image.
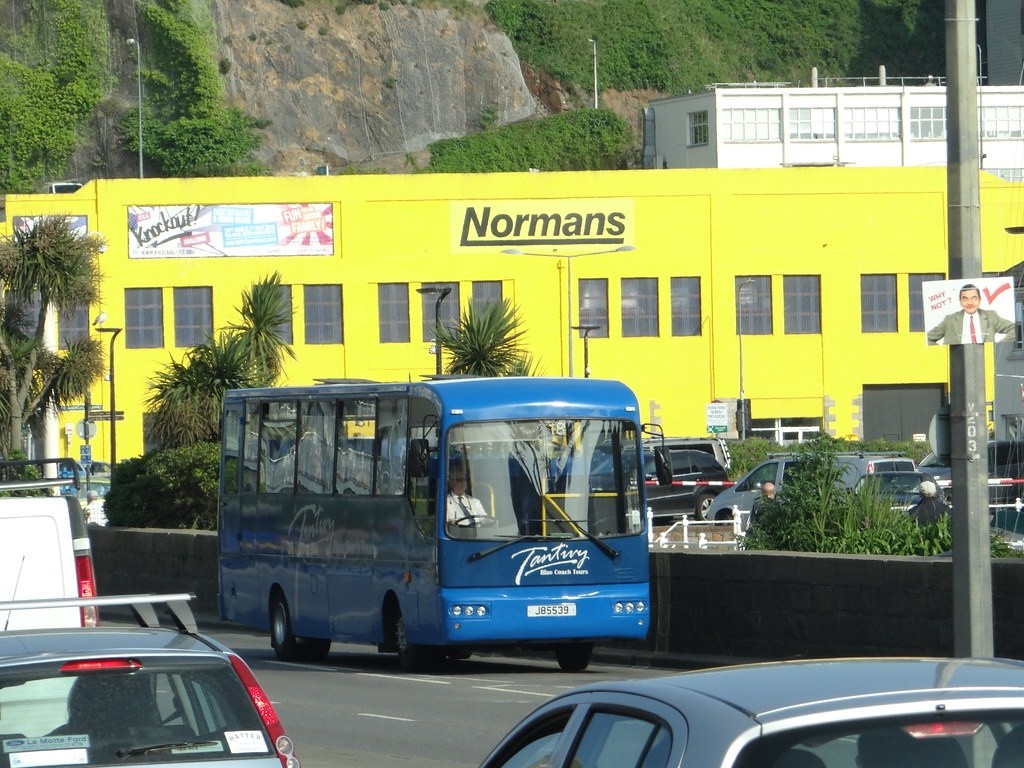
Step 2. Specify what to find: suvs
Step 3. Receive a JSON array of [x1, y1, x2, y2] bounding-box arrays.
[[0, 459, 102, 630], [0, 592, 301, 768], [587, 437, 735, 527], [708, 441, 1023, 528]]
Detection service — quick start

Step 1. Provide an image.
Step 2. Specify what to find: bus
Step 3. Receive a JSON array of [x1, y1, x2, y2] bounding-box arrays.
[[217, 375, 673, 671]]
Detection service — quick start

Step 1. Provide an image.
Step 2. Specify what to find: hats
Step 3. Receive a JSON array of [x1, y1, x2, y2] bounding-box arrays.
[[919, 480, 936, 493]]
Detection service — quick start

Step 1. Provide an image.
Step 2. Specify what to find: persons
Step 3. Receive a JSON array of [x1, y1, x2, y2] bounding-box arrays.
[[443, 472, 493, 530], [908, 481, 950, 527], [926, 285, 1017, 345], [746, 481, 779, 541]]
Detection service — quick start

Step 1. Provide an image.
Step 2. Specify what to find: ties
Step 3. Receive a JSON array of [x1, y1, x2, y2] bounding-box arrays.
[[970, 316, 977, 343], [458, 496, 475, 524]]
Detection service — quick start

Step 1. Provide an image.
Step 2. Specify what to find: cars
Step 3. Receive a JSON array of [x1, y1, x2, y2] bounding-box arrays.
[[480, 658, 1024, 768]]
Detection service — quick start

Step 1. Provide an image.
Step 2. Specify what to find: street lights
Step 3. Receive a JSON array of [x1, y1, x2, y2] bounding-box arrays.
[[96, 326, 123, 480], [416, 289, 451, 375], [573, 325, 600, 379], [588, 36, 599, 113], [125, 37, 144, 176], [500, 246, 636, 378], [737, 280, 754, 446]]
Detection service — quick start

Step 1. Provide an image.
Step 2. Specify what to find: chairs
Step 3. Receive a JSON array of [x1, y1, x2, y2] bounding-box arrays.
[[774, 720, 1024, 768], [67, 677, 160, 742]]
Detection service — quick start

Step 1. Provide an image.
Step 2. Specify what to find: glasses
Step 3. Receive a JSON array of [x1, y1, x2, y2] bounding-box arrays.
[[450, 478, 466, 482]]
